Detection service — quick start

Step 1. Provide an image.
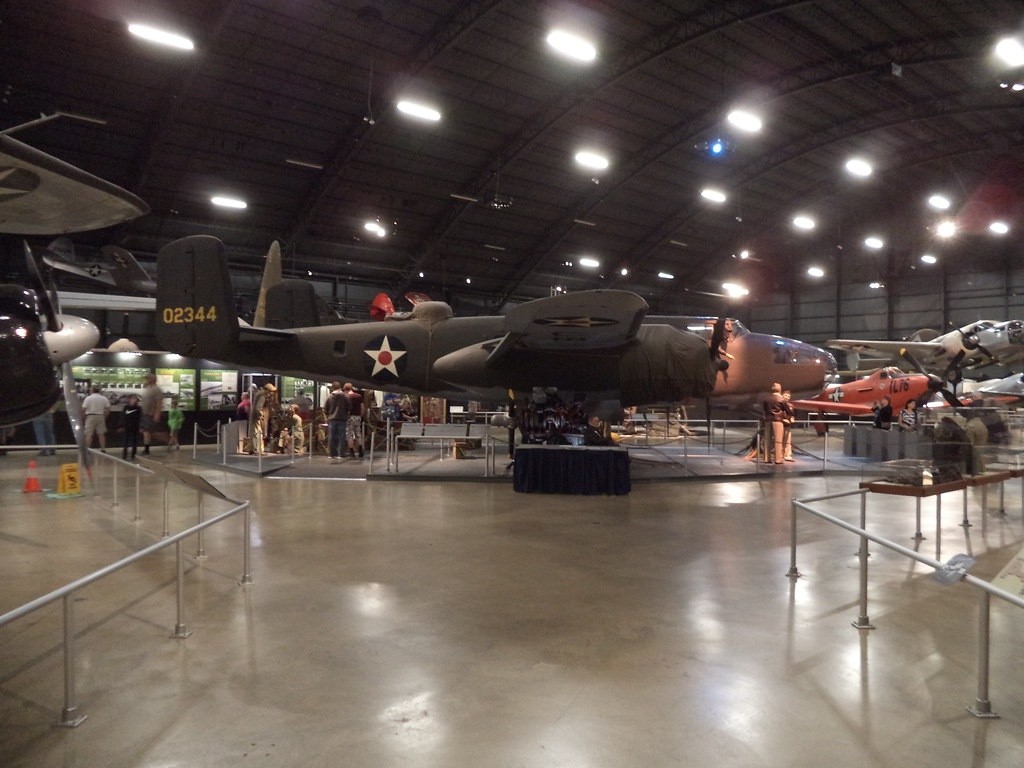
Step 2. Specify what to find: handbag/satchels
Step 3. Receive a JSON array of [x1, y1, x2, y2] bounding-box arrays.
[[881, 421, 890, 430]]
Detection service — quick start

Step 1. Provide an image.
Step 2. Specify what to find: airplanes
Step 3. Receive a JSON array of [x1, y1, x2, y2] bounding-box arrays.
[[788, 348, 969, 437], [926, 372, 1024, 408], [0, 238, 96, 483], [151, 234, 837, 459], [826, 320, 1023, 395]]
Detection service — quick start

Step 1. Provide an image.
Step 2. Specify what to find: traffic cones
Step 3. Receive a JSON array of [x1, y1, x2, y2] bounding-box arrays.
[[23, 460, 41, 493]]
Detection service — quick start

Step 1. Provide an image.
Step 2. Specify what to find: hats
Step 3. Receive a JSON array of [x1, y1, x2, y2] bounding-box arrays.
[[265, 383, 277, 391], [146, 373, 157, 380]]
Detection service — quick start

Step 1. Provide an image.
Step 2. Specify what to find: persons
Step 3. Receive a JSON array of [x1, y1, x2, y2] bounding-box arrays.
[[166, 400, 185, 452], [236, 382, 330, 460], [763, 383, 794, 464], [383, 391, 420, 422], [322, 382, 352, 461], [33, 407, 57, 456], [80, 384, 110, 453], [931, 398, 1006, 474], [897, 399, 922, 433], [583, 416, 612, 446], [781, 390, 796, 461], [139, 373, 170, 454], [341, 382, 365, 457], [871, 396, 893, 431], [117, 394, 142, 461]]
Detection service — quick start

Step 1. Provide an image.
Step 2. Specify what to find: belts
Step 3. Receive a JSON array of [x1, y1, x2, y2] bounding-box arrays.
[[85, 414, 98, 415]]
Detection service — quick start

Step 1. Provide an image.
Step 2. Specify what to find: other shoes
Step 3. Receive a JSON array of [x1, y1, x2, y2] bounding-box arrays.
[[328, 455, 338, 458], [358, 452, 364, 457], [347, 453, 355, 457], [37, 452, 55, 456], [100, 449, 106, 453], [338, 456, 351, 459]]
